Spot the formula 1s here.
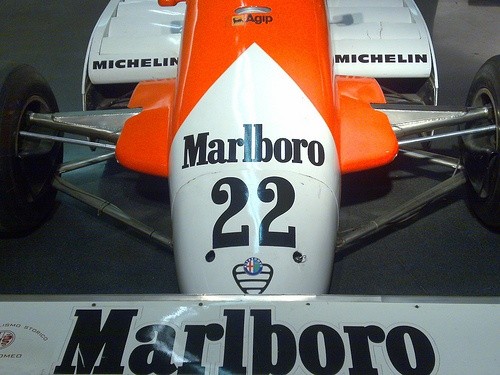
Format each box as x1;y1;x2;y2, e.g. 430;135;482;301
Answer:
1;0;499;374
